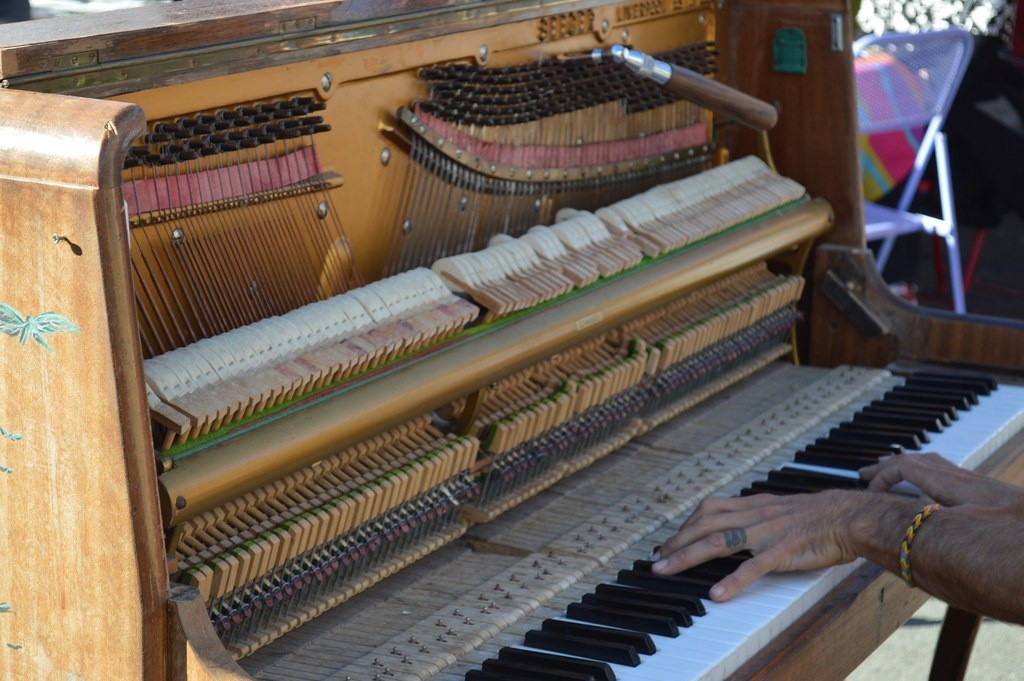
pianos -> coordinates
[1,1,1024,679]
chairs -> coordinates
[854,25,976,312]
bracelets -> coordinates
[899,506,938,600]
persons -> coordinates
[650,452,1024,626]
